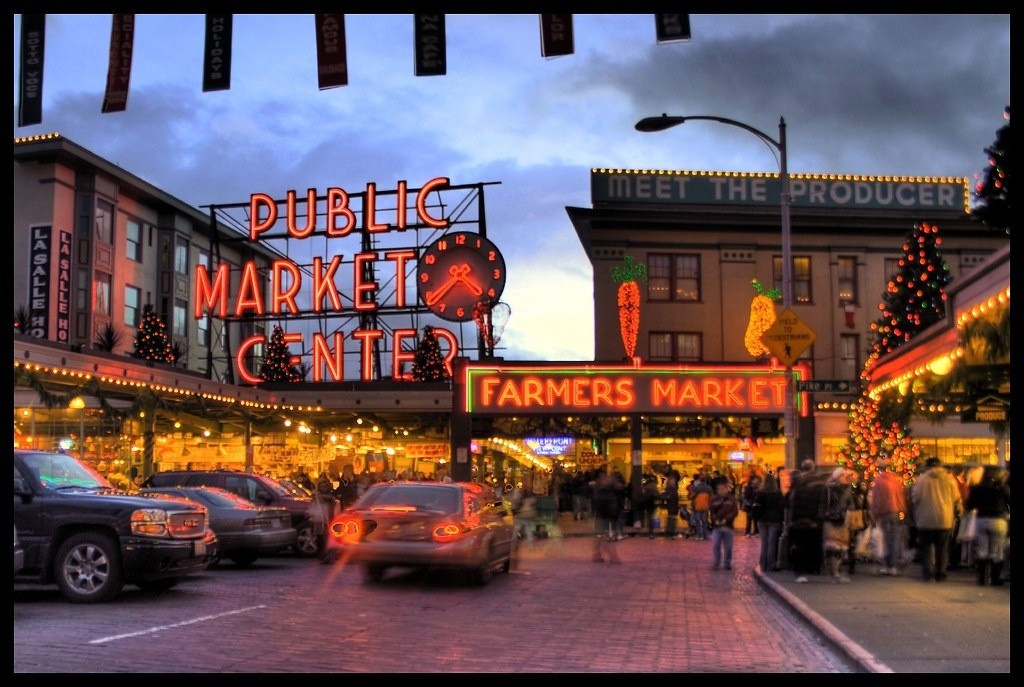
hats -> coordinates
[832,467,846,480]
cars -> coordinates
[138,486,298,570]
[329,483,514,589]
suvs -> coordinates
[15,450,217,603]
[140,470,343,556]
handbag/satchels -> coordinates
[956,509,977,542]
[855,524,886,560]
[846,508,864,530]
[823,523,849,552]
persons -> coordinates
[550,462,862,583]
[910,458,1011,586]
[872,459,904,575]
[300,465,448,535]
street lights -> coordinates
[634,112,793,470]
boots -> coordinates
[990,559,1004,586]
[976,557,989,586]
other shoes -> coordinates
[794,574,808,583]
[722,562,732,571]
[710,565,719,571]
[935,573,947,582]
[878,566,903,577]
[923,574,932,581]
[833,572,840,578]
